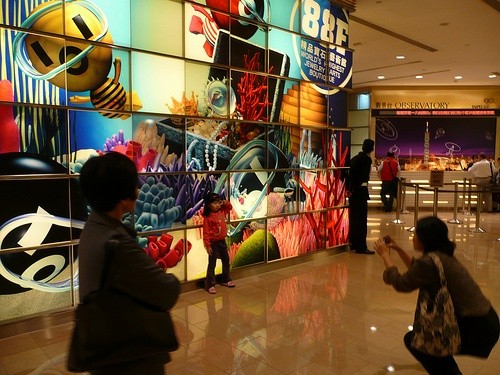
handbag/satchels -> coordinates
[67,234,180,372]
[412,251,461,355]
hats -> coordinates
[363,139,374,150]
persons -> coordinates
[378,151,400,213]
[373,216,500,375]
[203,192,236,294]
[346,139,375,254]
[77,150,179,375]
[468,153,500,212]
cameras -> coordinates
[383,235,390,245]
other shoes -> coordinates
[209,287,216,293]
[356,249,375,254]
[222,281,235,286]
[350,245,356,250]
